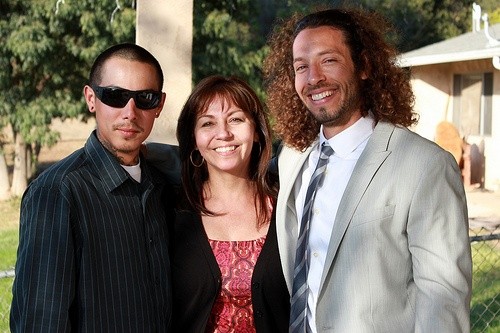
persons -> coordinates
[266,7,472,333]
[177,75,292,333]
[10,44,184,333]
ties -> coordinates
[289,140,334,333]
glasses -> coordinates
[92,84,162,109]
